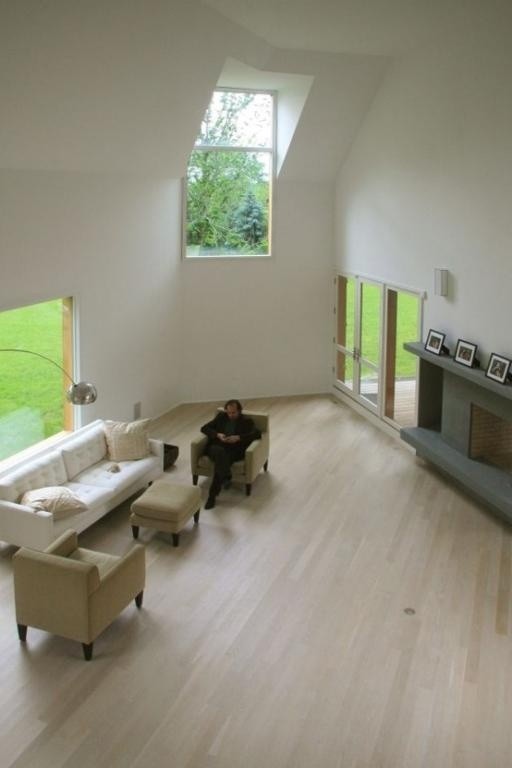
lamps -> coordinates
[434,266,449,298]
[1,348,102,406]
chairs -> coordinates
[10,528,148,662]
[189,403,271,496]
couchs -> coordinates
[0,418,178,552]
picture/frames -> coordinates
[451,337,478,368]
[424,328,446,356]
[484,352,511,384]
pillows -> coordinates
[102,416,154,464]
[19,487,90,519]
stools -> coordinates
[130,479,202,546]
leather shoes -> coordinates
[205,496,215,509]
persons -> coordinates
[199,399,261,510]
